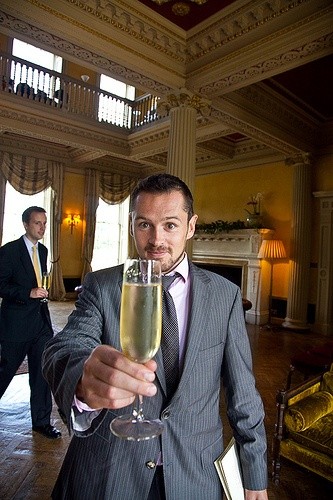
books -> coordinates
[214,436,244,500]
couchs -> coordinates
[270,364,333,486]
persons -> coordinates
[0,206,62,439]
[40,174,269,500]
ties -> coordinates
[32,246,42,288]
[156,274,179,398]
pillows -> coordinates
[284,391,333,432]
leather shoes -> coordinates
[32,424,61,439]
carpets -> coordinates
[14,299,76,374]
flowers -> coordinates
[244,192,264,215]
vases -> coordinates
[244,215,263,227]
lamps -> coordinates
[81,75,90,82]
[133,110,141,126]
[257,240,286,330]
[65,212,80,234]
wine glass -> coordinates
[109,258,164,442]
[40,271,51,302]
